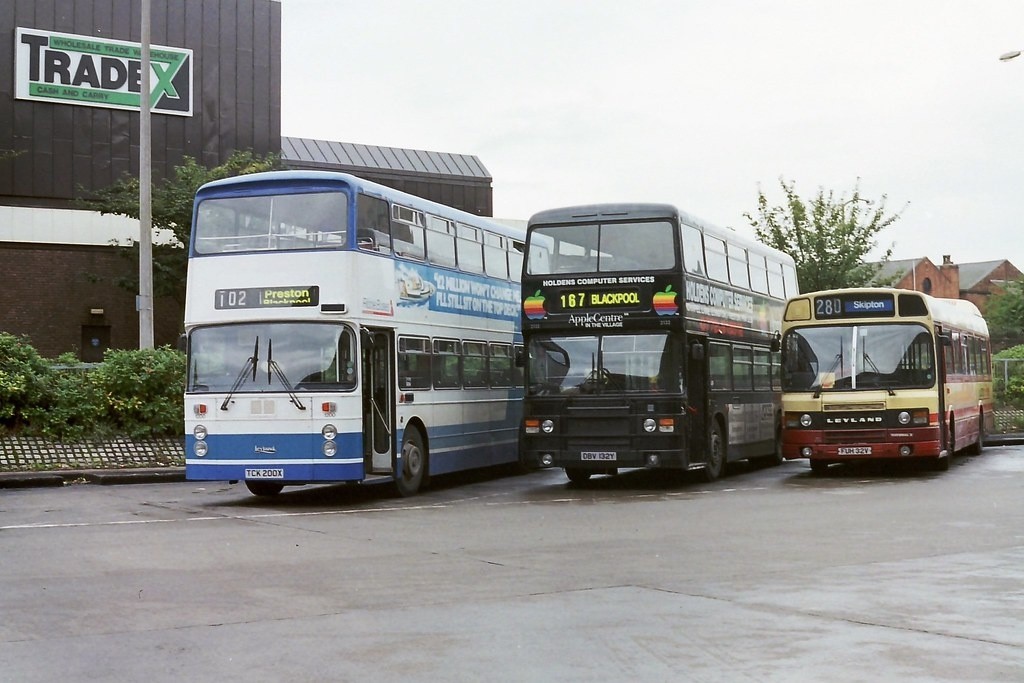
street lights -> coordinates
[840,197,876,239]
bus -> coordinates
[770,286,995,472]
[183,170,551,498]
[517,201,802,487]
